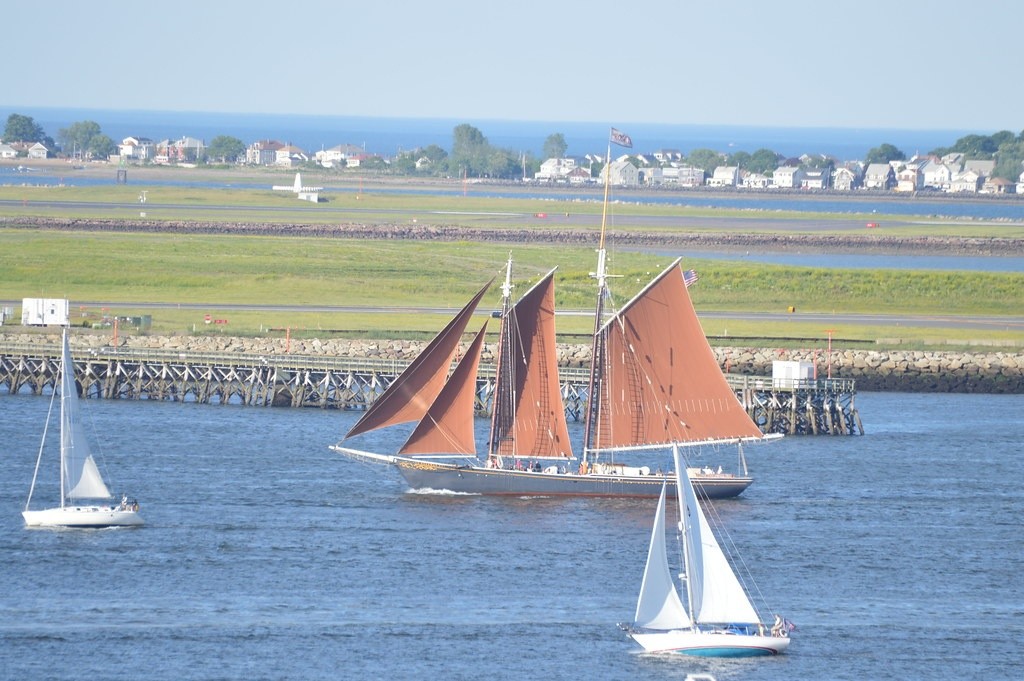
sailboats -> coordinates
[327,250,787,495]
[632,440,795,658]
[22,329,145,526]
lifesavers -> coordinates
[779,629,788,638]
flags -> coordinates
[609,127,632,148]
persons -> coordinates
[534,460,542,472]
[770,613,783,637]
